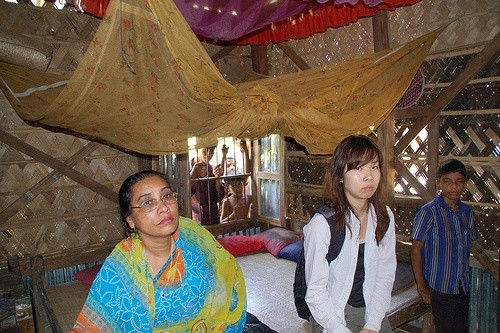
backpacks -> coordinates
[293,202,347,322]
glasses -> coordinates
[131,192,178,213]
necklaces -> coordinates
[146,236,174,298]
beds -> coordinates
[29,219,435,333]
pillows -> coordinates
[391,259,416,294]
[70,264,101,285]
[216,228,304,264]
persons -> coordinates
[293,135,397,333]
[71,170,247,333]
[189,135,252,225]
[411,159,500,333]
